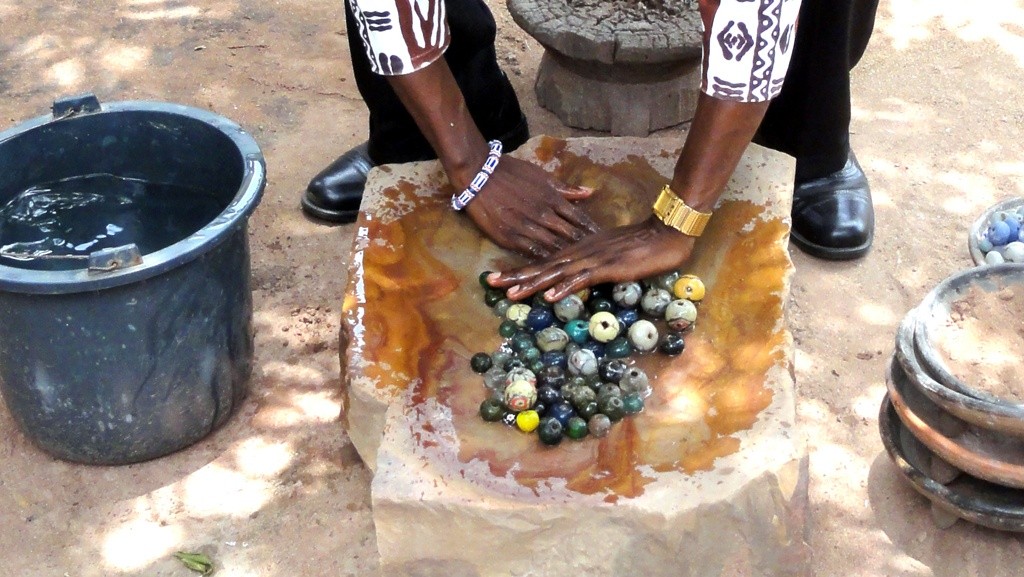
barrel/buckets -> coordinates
[0,93,265,468]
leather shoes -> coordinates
[790,148,874,258]
[301,140,374,223]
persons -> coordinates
[300,0,882,303]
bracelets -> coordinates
[450,139,504,211]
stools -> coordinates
[507,0,706,136]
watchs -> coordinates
[652,183,712,237]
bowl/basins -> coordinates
[876,195,1023,536]
